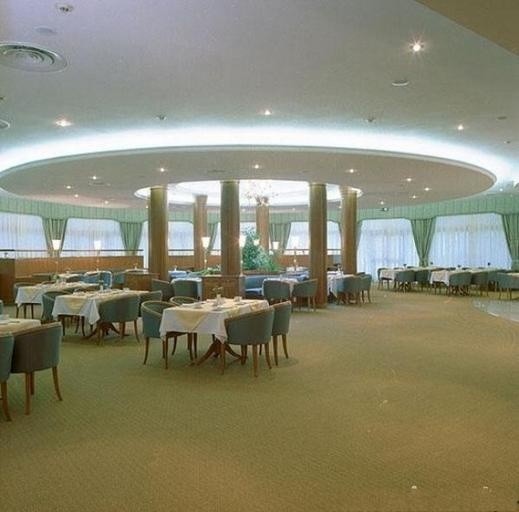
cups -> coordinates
[0,313,9,324]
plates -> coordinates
[182,292,248,311]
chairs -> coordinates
[169,295,200,357]
[222,306,272,377]
[11,263,318,326]
[261,300,292,366]
[326,263,519,307]
[1,332,17,421]
[140,300,173,366]
[11,322,64,416]
[82,294,139,346]
